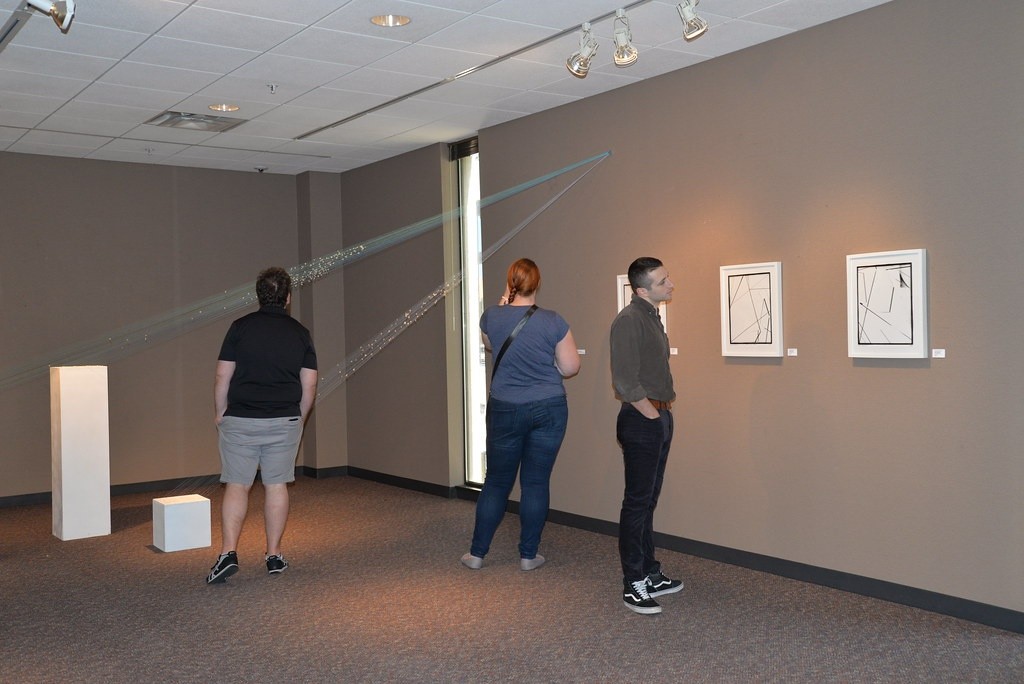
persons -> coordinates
[461,258,580,571]
[206,267,317,584]
[610,257,683,614]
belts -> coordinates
[649,400,672,410]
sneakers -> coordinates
[206,550,239,584]
[622,577,662,614]
[645,570,684,598]
[265,552,291,573]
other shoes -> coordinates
[521,554,545,570]
[461,552,483,569]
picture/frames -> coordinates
[616,274,667,333]
[720,262,783,358]
[846,248,928,358]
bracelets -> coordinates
[501,296,506,300]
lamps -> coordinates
[26,0,76,30]
[566,21,597,78]
[612,8,637,67]
[676,0,708,40]
